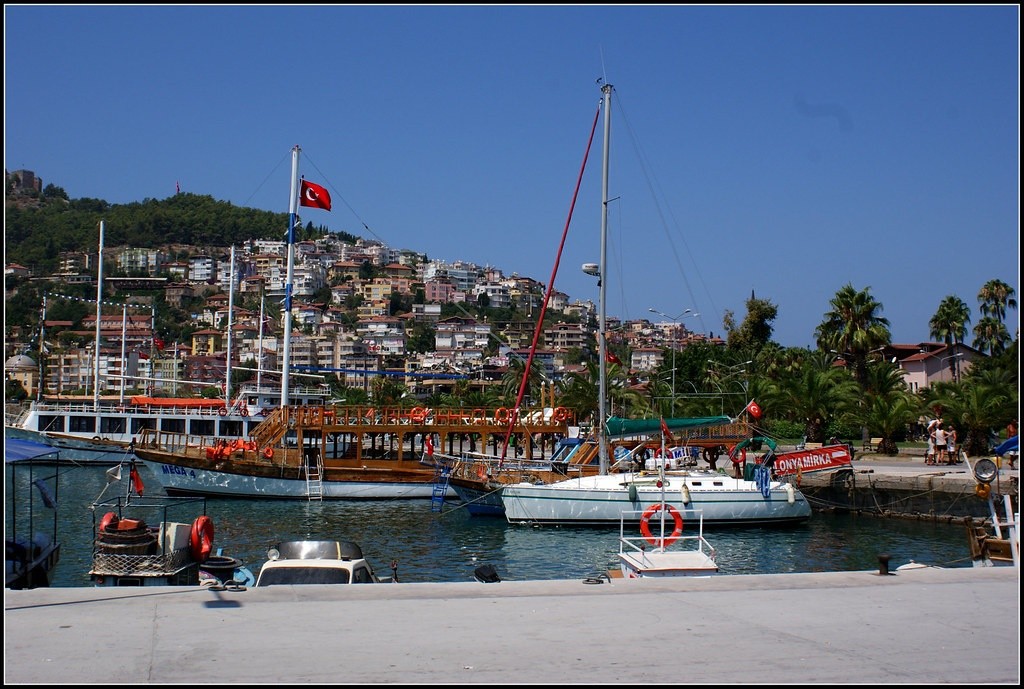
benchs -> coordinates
[924,444,962,463]
[804,442,823,450]
[862,437,884,452]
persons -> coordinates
[1007,418,1019,469]
[213,436,239,449]
[150,437,157,447]
[924,409,957,465]
[130,436,136,450]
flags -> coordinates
[131,470,144,493]
[139,349,151,360]
[605,349,623,367]
[661,419,676,440]
[747,401,762,418]
[298,177,331,210]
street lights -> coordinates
[920,350,964,382]
[649,308,701,418]
[708,360,753,392]
[657,369,677,383]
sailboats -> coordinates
[482,80,814,527]
[10,139,461,499]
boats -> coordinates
[254,539,398,587]
[433,413,751,516]
[608,408,722,579]
[87,437,257,587]
[6,439,63,591]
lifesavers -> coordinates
[102,437,110,442]
[93,436,102,446]
[729,445,746,463]
[702,448,720,463]
[190,516,214,565]
[633,449,650,466]
[410,406,426,423]
[640,504,681,547]
[495,407,509,423]
[218,407,228,417]
[264,447,274,457]
[655,448,674,466]
[261,408,269,417]
[555,406,568,421]
[240,408,248,417]
[472,409,486,423]
